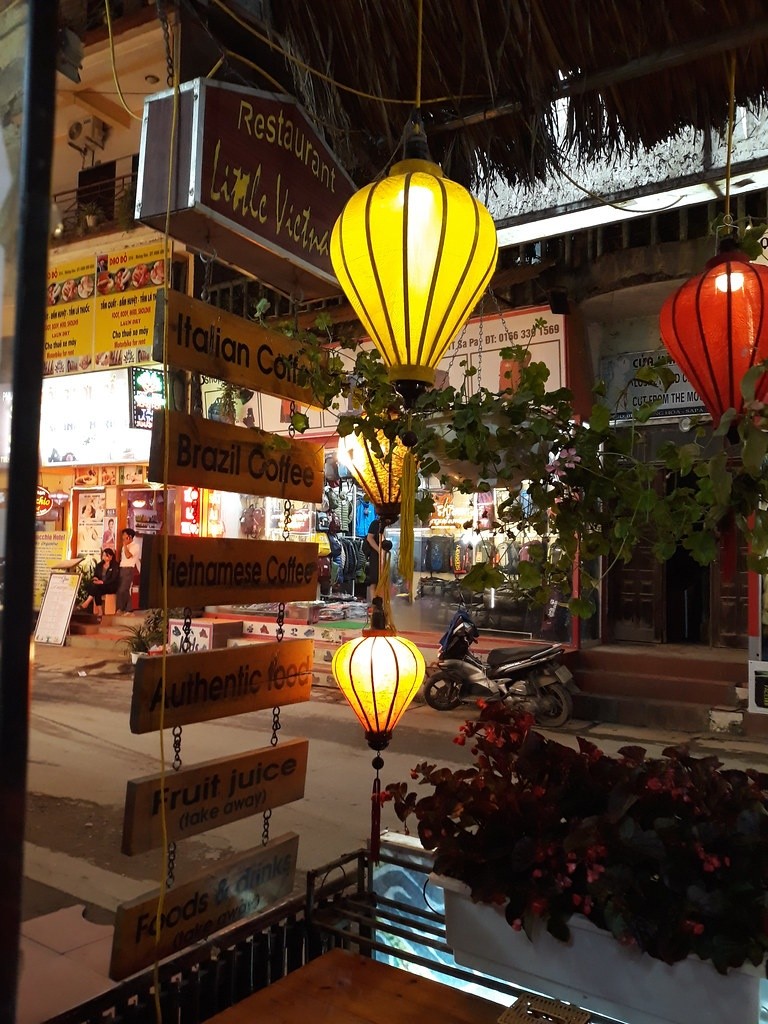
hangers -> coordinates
[336,536,367,542]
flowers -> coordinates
[388,701,767,974]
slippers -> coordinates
[96,613,103,624]
[76,605,88,611]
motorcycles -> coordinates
[422,592,580,727]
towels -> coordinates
[438,608,479,651]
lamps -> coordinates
[331,598,428,774]
[336,405,423,523]
[331,3,496,389]
[656,2,768,425]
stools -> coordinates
[93,594,116,615]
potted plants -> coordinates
[74,200,112,235]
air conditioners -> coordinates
[68,116,108,148]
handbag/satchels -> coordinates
[447,579,541,637]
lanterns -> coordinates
[330,158,499,605]
[331,610,425,866]
[659,249,768,444]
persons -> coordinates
[361,515,399,614]
[74,548,119,622]
[114,528,140,617]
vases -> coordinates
[424,854,763,1024]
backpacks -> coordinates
[327,532,342,557]
[329,557,340,586]
[518,540,541,563]
[311,532,331,556]
[324,451,340,481]
[338,460,351,478]
[316,510,329,531]
[315,494,329,512]
[326,510,340,533]
[325,488,339,509]
[317,557,332,584]
[497,540,518,574]
[239,504,255,534]
[423,536,449,573]
[450,534,472,574]
[208,397,236,425]
[519,490,533,518]
[475,537,495,563]
[546,538,565,567]
[252,507,265,538]
